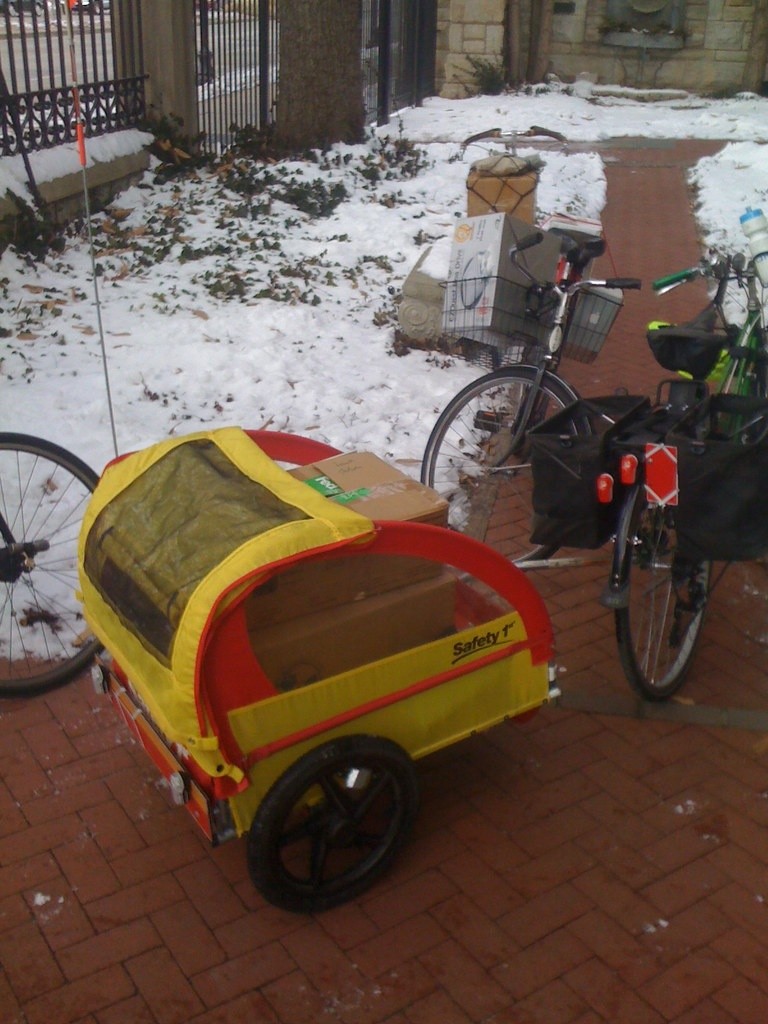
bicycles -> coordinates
[421,227,768,704]
[0,431,110,697]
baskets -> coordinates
[439,276,625,372]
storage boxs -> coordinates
[241,449,449,633]
[544,212,601,289]
[563,285,624,352]
[249,573,461,694]
[442,213,562,349]
[466,166,539,225]
